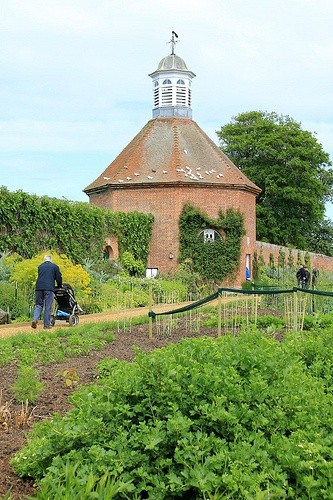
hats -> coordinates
[300,264,304,268]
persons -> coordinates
[296,263,319,289]
[67,289,84,312]
[246,267,251,281]
[31,254,64,329]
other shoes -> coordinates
[44,326,52,329]
[32,320,37,328]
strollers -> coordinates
[50,283,80,326]
